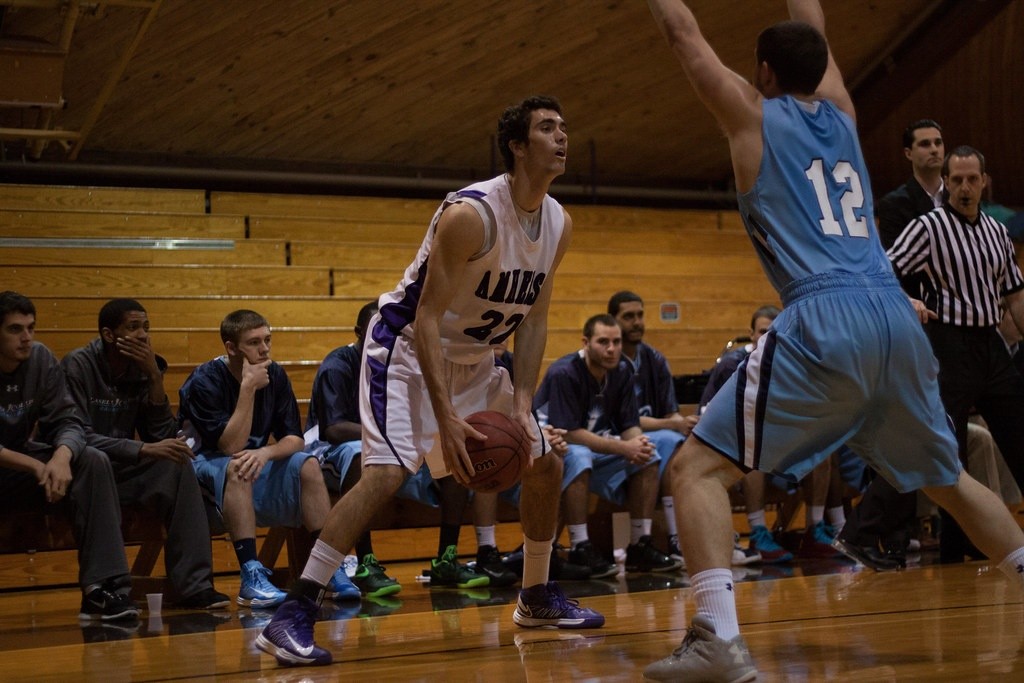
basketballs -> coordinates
[454,410,534,494]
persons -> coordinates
[0,289,139,621]
[61,298,233,611]
[608,290,765,565]
[176,310,369,609]
[644,1,1024,683]
[698,304,880,571]
[304,301,491,598]
[889,124,1024,566]
[479,332,568,587]
[537,313,686,579]
[254,95,610,666]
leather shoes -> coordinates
[837,531,901,570]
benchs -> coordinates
[0,185,784,589]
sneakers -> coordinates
[324,561,361,598]
[77,584,141,620]
[328,590,510,641]
[642,614,758,683]
[514,629,605,667]
[749,531,793,563]
[473,556,517,586]
[354,553,402,596]
[798,522,848,557]
[624,536,682,571]
[235,560,287,608]
[177,586,231,609]
[667,538,686,571]
[731,541,762,565]
[254,595,332,666]
[513,581,604,628]
[421,545,489,589]
[77,619,142,651]
[506,539,620,579]
